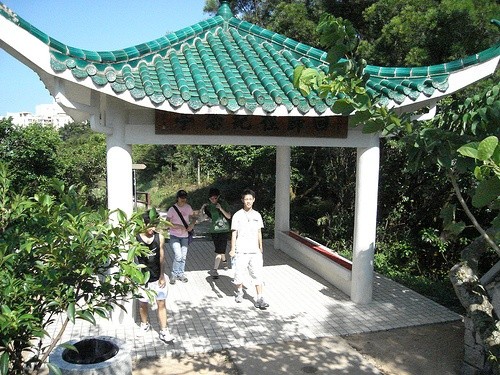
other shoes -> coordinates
[212,269,219,279]
[159,328,175,342]
[223,260,228,269]
[254,298,269,308]
[177,274,188,283]
[136,322,150,337]
[169,275,176,284]
[235,292,243,303]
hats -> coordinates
[178,190,188,199]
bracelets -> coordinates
[191,224,194,228]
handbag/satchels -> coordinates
[187,229,194,244]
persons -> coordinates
[229,189,269,308]
[131,210,175,343]
[164,190,196,284]
[199,188,232,277]
